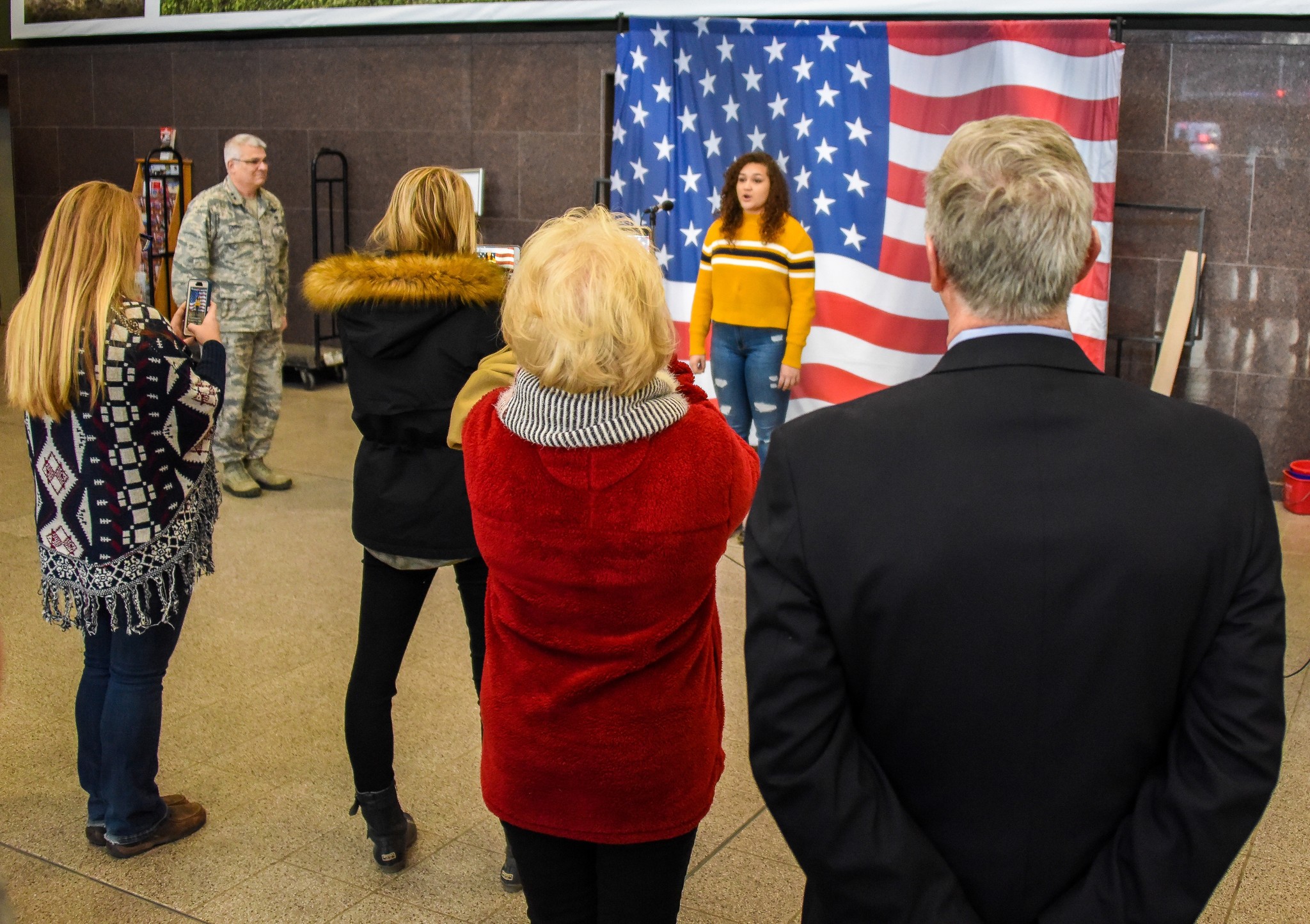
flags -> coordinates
[612,18,1125,452]
[189,287,207,312]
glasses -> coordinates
[233,158,268,165]
[139,232,154,251]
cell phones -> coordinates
[183,278,214,336]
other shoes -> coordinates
[729,522,745,545]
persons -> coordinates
[195,295,201,318]
[6,180,226,858]
[302,166,527,893]
[691,152,814,543]
[171,133,293,497]
[741,119,1286,924]
[460,204,758,924]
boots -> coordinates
[501,842,524,894]
[349,782,417,874]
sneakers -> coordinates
[245,458,292,490]
[222,460,262,498]
[107,802,207,859]
[86,794,188,847]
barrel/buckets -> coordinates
[1283,461,1310,515]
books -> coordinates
[135,163,182,303]
[160,126,176,161]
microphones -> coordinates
[643,201,673,214]
